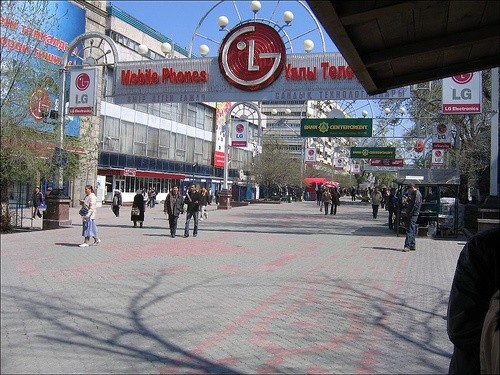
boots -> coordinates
[139,222,143,228]
[132,222,137,228]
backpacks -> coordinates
[479,288,500,375]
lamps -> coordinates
[362,110,368,117]
[161,41,171,59]
[138,44,148,61]
[251,0,261,17]
[285,109,291,118]
[400,108,405,116]
[283,10,293,27]
[218,15,229,33]
[272,108,277,117]
[384,107,391,117]
[198,45,208,57]
[303,39,315,53]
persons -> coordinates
[131,186,158,228]
[371,187,383,218]
[111,188,123,217]
[184,184,201,237]
[281,184,304,202]
[402,182,422,252]
[164,184,184,238]
[388,188,396,229]
[447,227,500,375]
[79,184,98,246]
[316,182,358,215]
[370,185,398,210]
[199,186,220,219]
[29,185,53,219]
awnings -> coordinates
[308,0,500,95]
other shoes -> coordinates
[200,218,203,221]
[92,240,101,245]
[79,243,89,247]
[184,233,189,237]
[172,232,175,238]
[37,217,42,219]
[193,234,196,237]
[204,212,208,219]
[403,248,409,252]
[32,217,34,220]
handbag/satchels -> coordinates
[132,208,140,215]
[79,208,89,216]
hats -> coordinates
[35,186,40,189]
[48,187,52,191]
